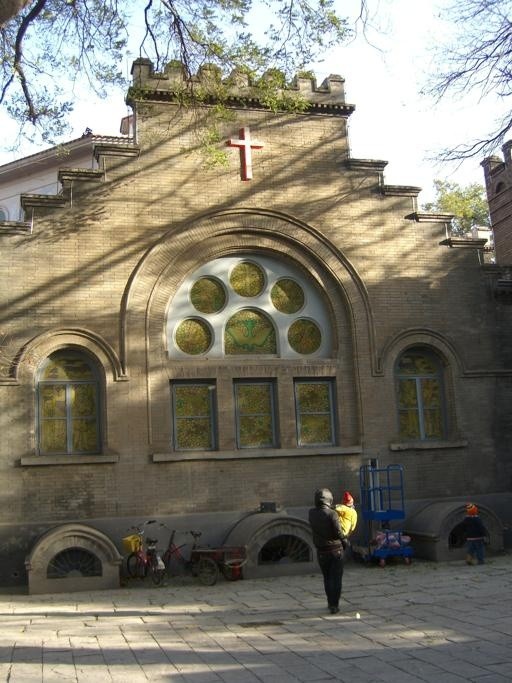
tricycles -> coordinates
[157,522,247,587]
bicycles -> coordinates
[123,518,167,584]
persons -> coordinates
[332,488,357,535]
[460,501,487,566]
[309,486,350,614]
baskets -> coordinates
[122,535,143,552]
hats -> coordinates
[343,492,354,507]
[466,503,478,515]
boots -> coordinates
[466,554,474,565]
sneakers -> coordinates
[331,607,339,612]
